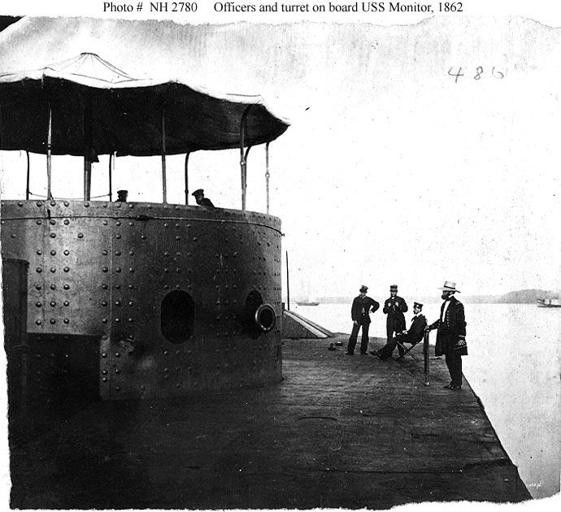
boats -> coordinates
[294,299,320,307]
[535,297,560,309]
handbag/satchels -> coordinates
[452,344,468,356]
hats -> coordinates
[359,285,368,293]
[413,302,422,309]
[117,190,127,194]
[191,189,204,196]
[437,280,461,294]
[389,285,398,292]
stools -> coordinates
[394,338,420,362]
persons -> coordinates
[371,302,427,361]
[425,282,467,390]
[384,286,408,359]
[115,190,128,202]
[345,286,380,356]
[193,189,216,208]
